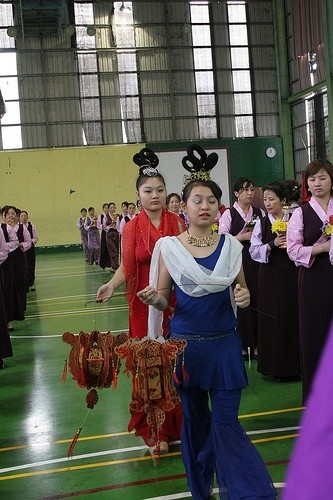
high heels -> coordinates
[241,347,249,361]
[252,347,259,360]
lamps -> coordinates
[119,2,132,14]
[183,24,191,34]
[64,26,74,35]
[7,27,17,37]
[86,26,96,36]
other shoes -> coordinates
[148,442,169,455]
[0,358,4,369]
[7,323,14,332]
[31,285,35,291]
[110,267,115,274]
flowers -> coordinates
[323,215,333,237]
[271,219,287,248]
[212,224,217,232]
[244,214,259,232]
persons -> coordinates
[135,144,278,500]
[0,205,39,369]
[77,159,333,405]
[96,147,187,456]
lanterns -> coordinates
[61,331,130,458]
[114,335,188,465]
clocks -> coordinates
[266,147,276,157]
